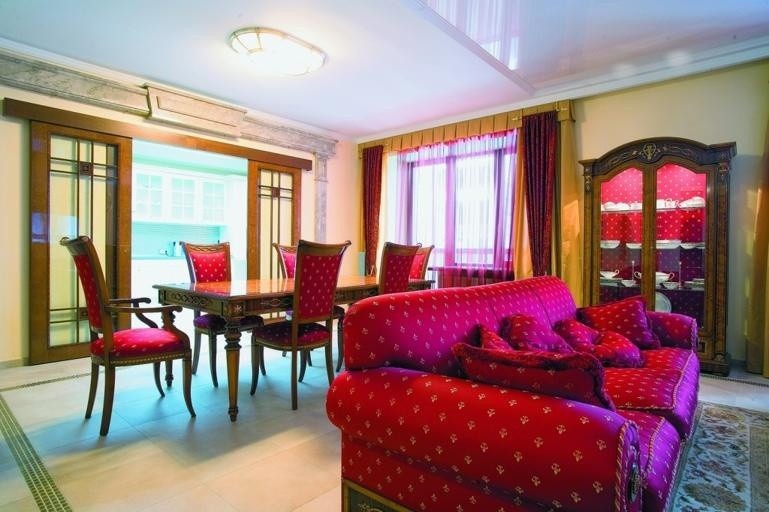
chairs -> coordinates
[272,239,351,373]
[57,234,200,436]
[244,239,337,411]
[177,237,268,390]
[410,244,438,281]
[377,240,423,295]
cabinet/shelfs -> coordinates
[577,136,738,376]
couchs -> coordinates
[323,274,702,512]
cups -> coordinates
[679,196,706,207]
[601,198,680,211]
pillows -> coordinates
[453,292,665,413]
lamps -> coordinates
[227,25,326,76]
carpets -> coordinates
[659,400,769,512]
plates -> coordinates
[655,292,672,314]
[599,278,623,282]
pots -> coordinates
[634,270,674,286]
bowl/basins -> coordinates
[621,279,636,287]
[627,243,642,248]
[600,241,621,249]
[684,278,704,290]
[600,270,618,279]
[656,239,680,249]
[662,281,679,289]
[682,244,695,250]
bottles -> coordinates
[358,251,365,277]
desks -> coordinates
[149,272,439,424]
